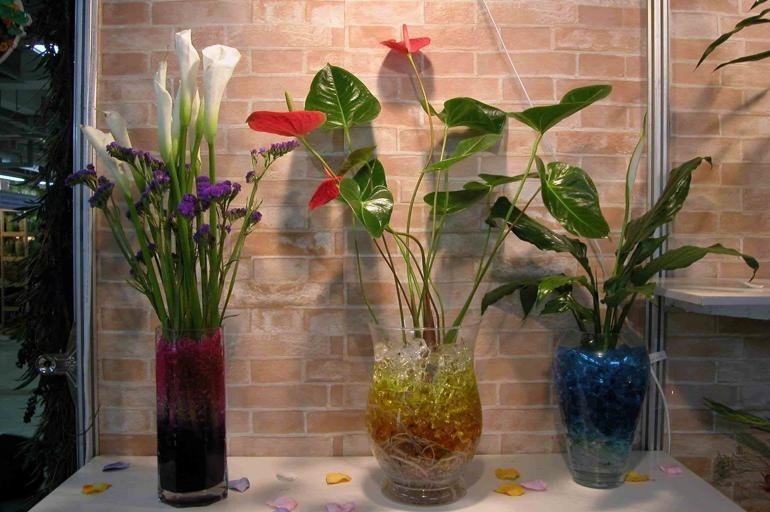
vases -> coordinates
[369,318,478,506]
[151,325,229,504]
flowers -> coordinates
[64,29,310,324]
[245,22,610,343]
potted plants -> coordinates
[463,92,757,494]
